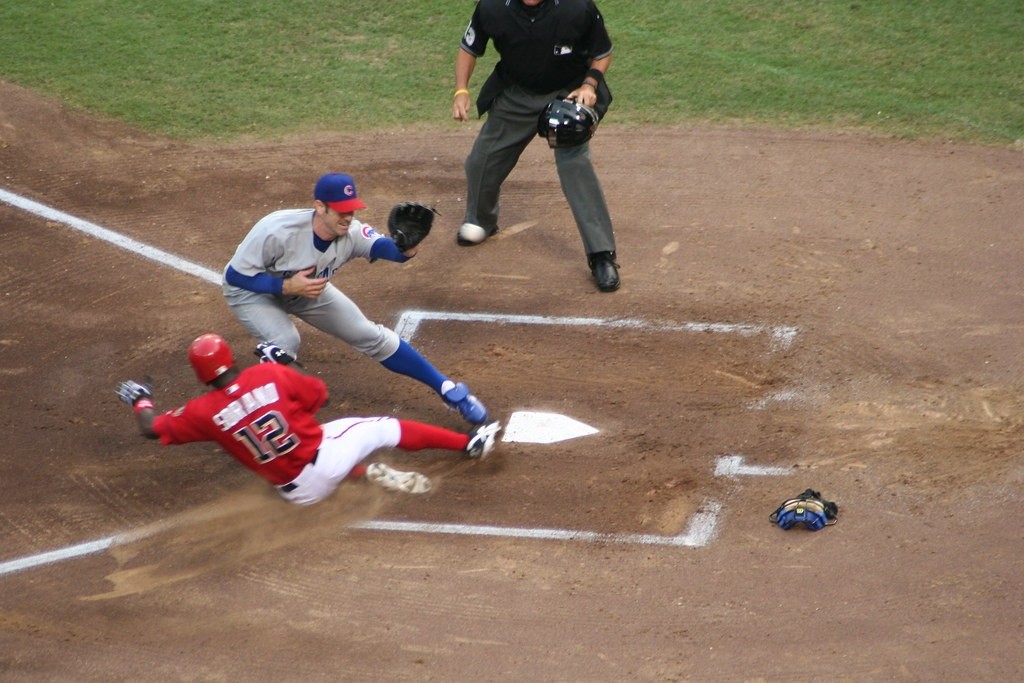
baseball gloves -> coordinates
[769,488,838,532]
[387,202,433,250]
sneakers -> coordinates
[442,382,486,424]
[464,420,501,460]
[366,463,432,494]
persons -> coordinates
[113,333,504,507]
[222,172,487,426]
[452,0,620,291]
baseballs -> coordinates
[459,223,486,244]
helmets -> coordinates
[537,96,599,149]
[189,334,236,386]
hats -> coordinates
[314,171,367,213]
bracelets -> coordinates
[134,399,154,413]
[585,68,603,83]
[582,81,597,89]
[454,89,470,98]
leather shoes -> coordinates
[457,223,498,246]
[591,255,620,291]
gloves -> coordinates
[116,380,152,406]
[253,341,294,365]
